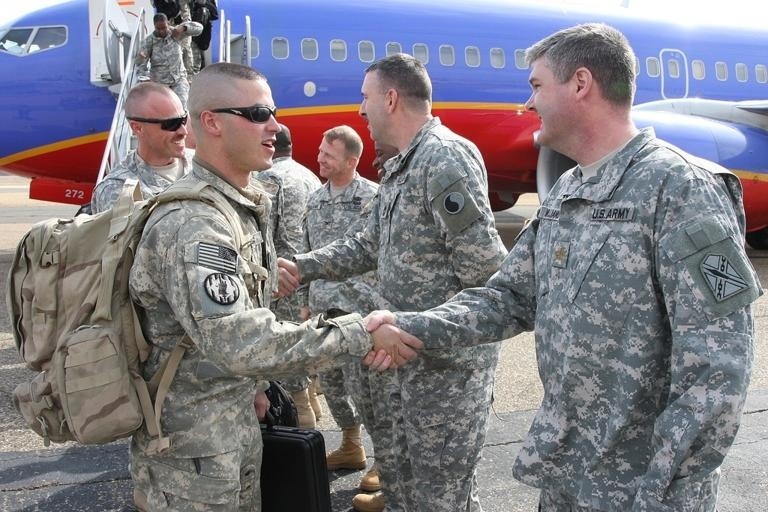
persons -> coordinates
[126,63,422,512]
[352,138,400,512]
[276,52,509,512]
[256,124,323,431]
[134,0,218,147]
[293,125,381,492]
[90,82,300,301]
[362,22,765,512]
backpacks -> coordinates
[5,181,243,446]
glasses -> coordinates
[126,111,188,131]
[211,106,276,124]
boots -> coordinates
[308,377,323,417]
[292,389,317,430]
[327,426,366,471]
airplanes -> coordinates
[0,0,768,251]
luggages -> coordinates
[261,381,331,512]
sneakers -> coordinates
[352,492,385,512]
[361,464,381,491]
